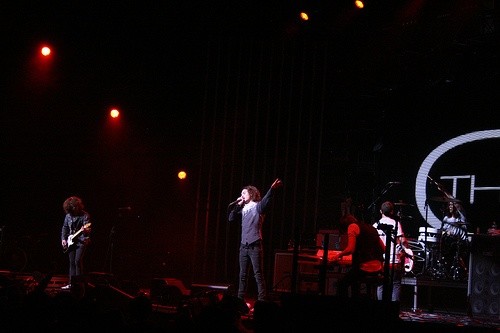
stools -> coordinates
[364,276,384,303]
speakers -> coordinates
[465,235,500,318]
[273,253,374,299]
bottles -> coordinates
[492,221,496,233]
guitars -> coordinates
[63,223,91,255]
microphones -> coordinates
[230,199,242,205]
[389,182,399,184]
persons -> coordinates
[372,202,405,301]
[441,201,467,236]
[333,214,382,296]
[228,179,282,302]
[61,196,92,289]
[0,291,244,333]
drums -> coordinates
[417,227,442,243]
[403,241,426,277]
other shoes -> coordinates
[61,284,72,289]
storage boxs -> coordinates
[317,234,338,247]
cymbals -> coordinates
[427,197,463,202]
[394,203,415,206]
[450,222,470,225]
